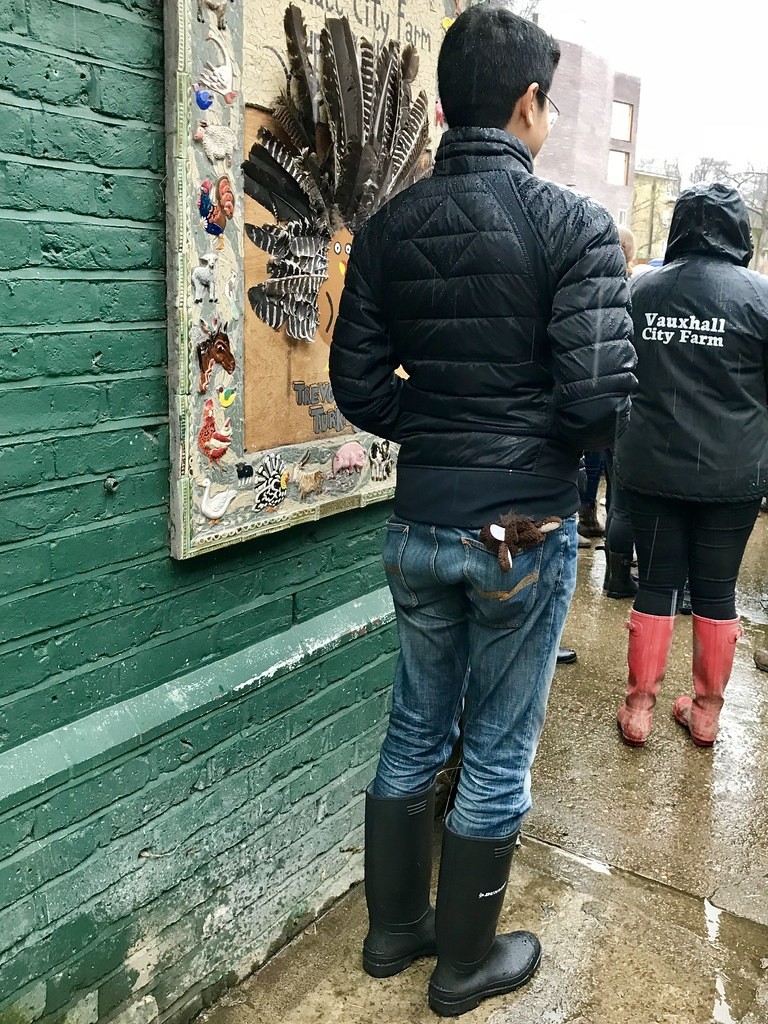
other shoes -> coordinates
[754,649,768,674]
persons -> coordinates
[328,2,639,1017]
[610,182,768,746]
[553,224,768,672]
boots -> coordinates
[364,776,434,977]
[576,467,605,539]
[423,808,540,1018]
[615,606,675,746]
[602,546,639,600]
[672,611,745,749]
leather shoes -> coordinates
[555,649,582,666]
[578,532,591,550]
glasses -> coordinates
[535,84,565,135]
